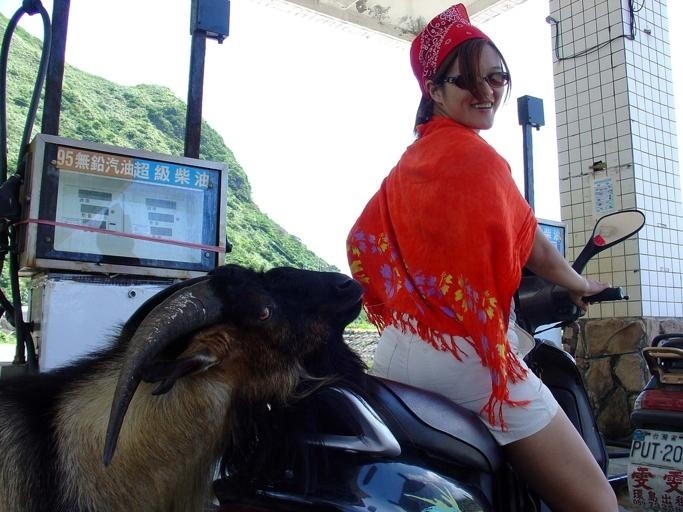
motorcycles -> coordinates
[627,332,683,512]
[208,209,647,512]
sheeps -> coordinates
[0,263,363,512]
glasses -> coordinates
[442,71,510,88]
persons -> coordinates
[346,1,620,512]
